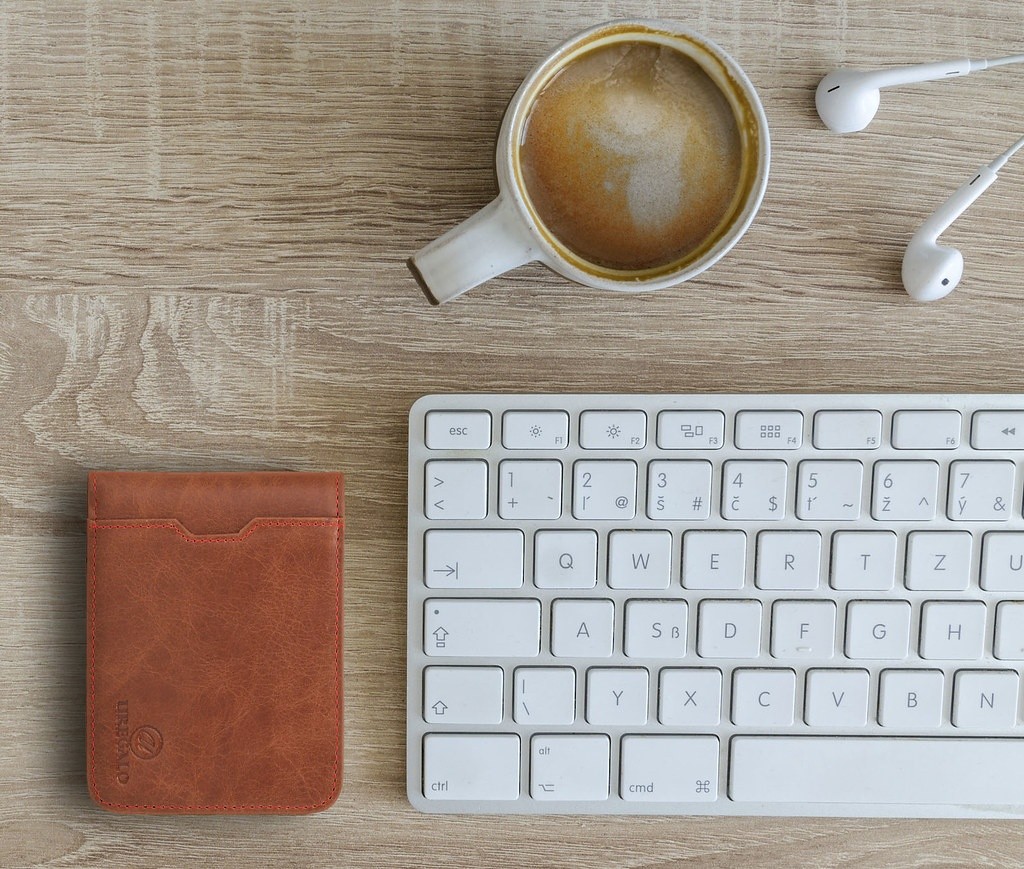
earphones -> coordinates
[901,153,1007,301]
[815,57,988,132]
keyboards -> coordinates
[405,395,1024,823]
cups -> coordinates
[399,13,774,308]
